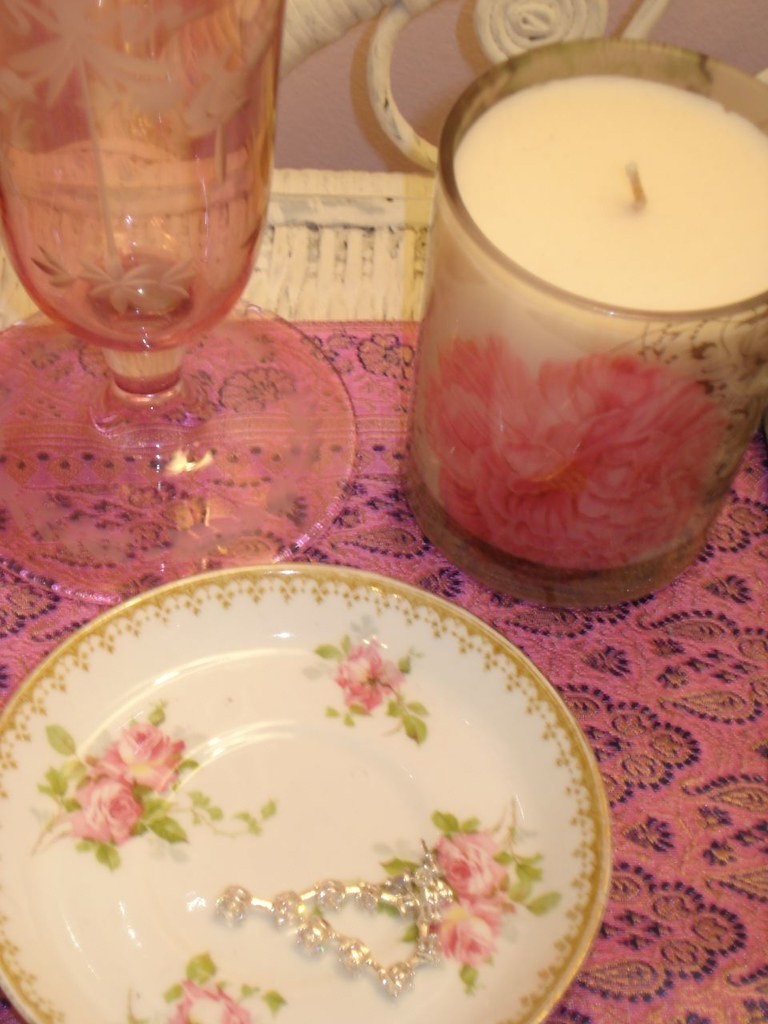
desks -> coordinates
[0,169,768,1022]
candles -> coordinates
[415,74,767,577]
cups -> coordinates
[405,37,767,607]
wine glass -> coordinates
[0,0,362,605]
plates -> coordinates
[0,564,616,1024]
